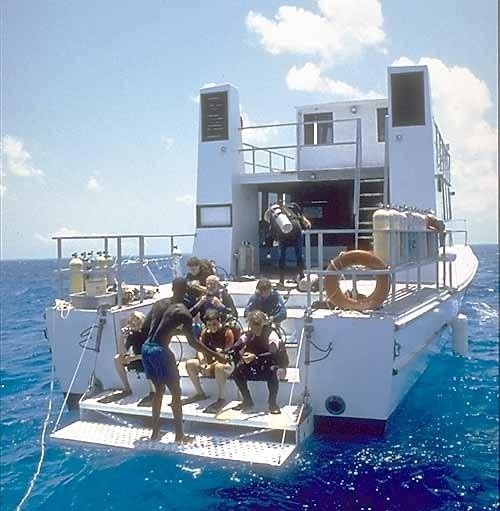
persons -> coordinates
[231,309,287,416]
[185,309,236,415]
[189,275,239,339]
[97,311,158,409]
[139,277,231,448]
[269,200,312,289]
[242,279,288,331]
[184,255,216,307]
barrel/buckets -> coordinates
[84,279,107,296]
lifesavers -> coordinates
[323,249,391,310]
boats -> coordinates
[42,62,481,471]
